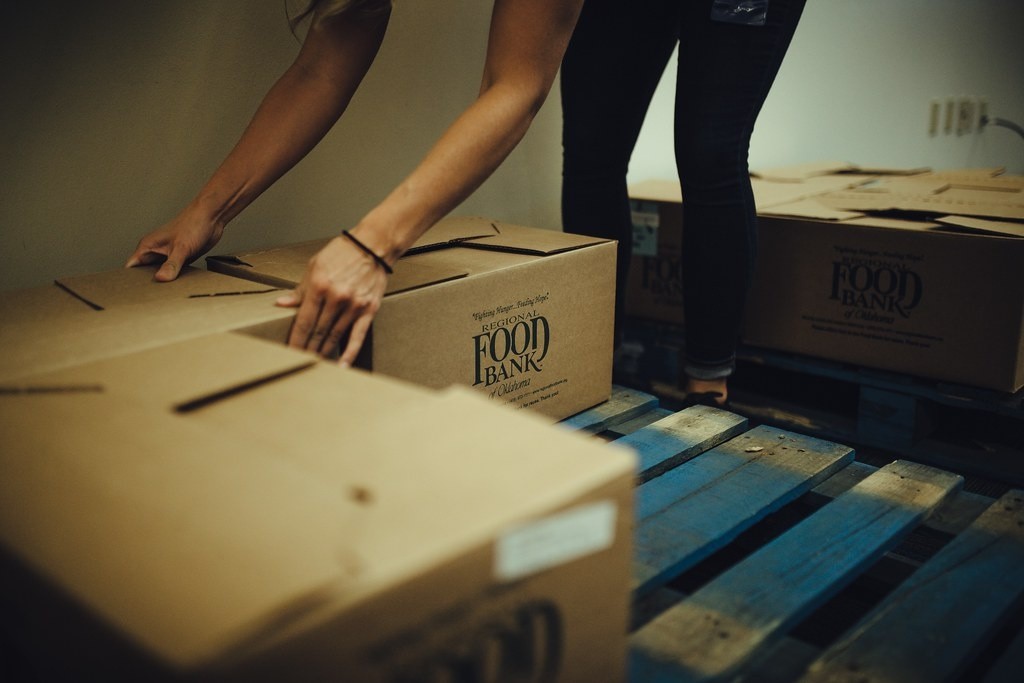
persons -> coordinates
[127,0,807,408]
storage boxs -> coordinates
[0,330,639,683]
[623,163,1024,394]
[0,262,340,384]
[204,212,619,420]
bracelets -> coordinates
[343,230,394,274]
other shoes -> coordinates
[681,391,729,410]
